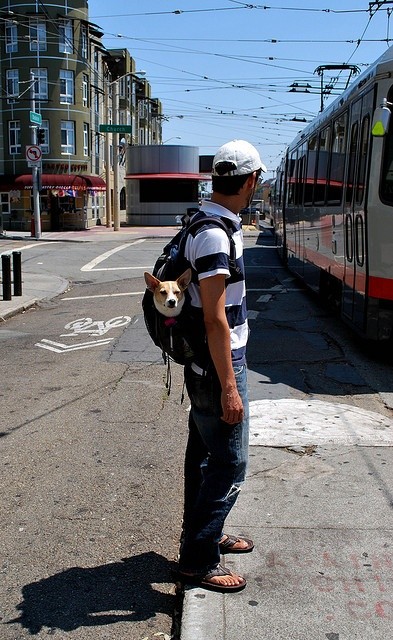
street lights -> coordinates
[162,136,180,147]
[155,115,184,144]
[105,70,146,231]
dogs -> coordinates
[141,262,193,320]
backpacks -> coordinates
[141,210,237,405]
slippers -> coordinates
[179,564,247,592]
[219,534,253,553]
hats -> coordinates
[212,140,267,176]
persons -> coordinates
[175,138,266,593]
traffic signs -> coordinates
[26,146,41,163]
[30,111,43,126]
[99,124,131,135]
